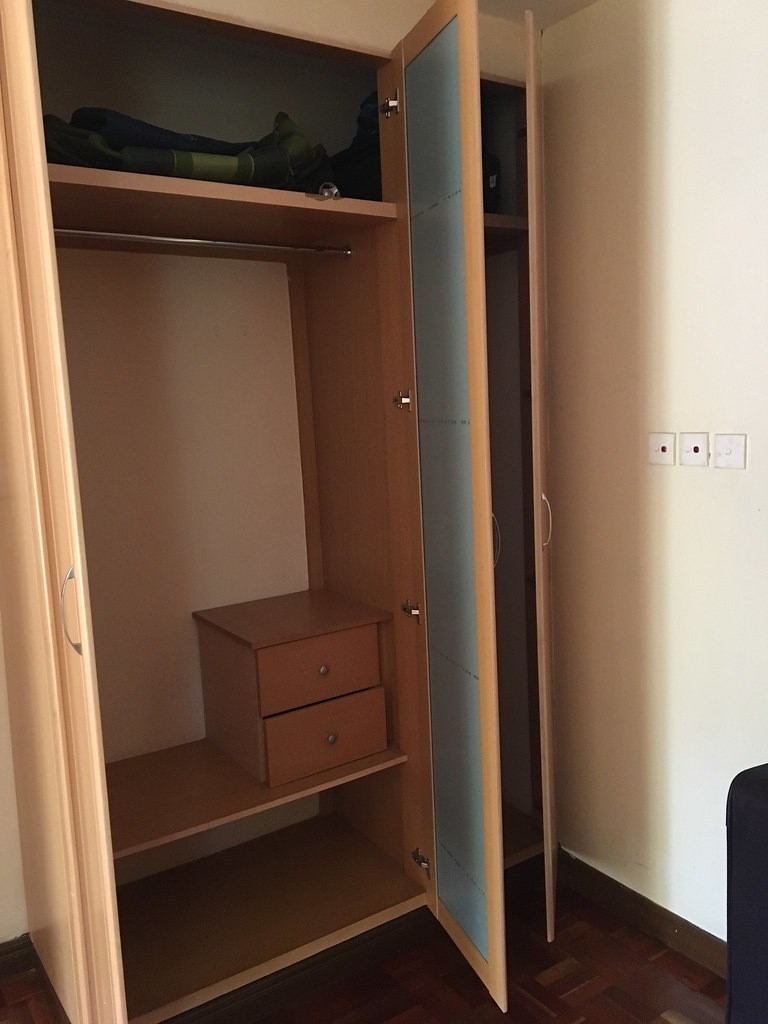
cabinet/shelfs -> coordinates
[0,0,513,1024]
[185,583,387,785]
